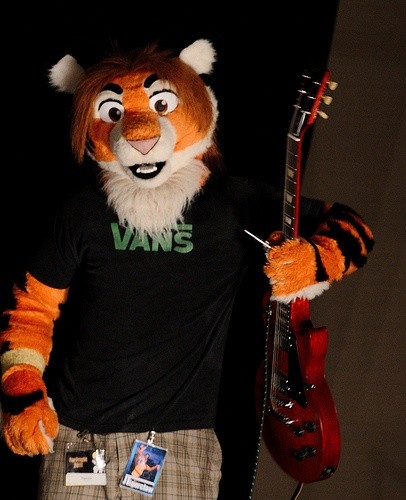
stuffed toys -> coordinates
[0,39,375,500]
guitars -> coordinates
[261,67,339,485]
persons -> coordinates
[131,445,160,477]
